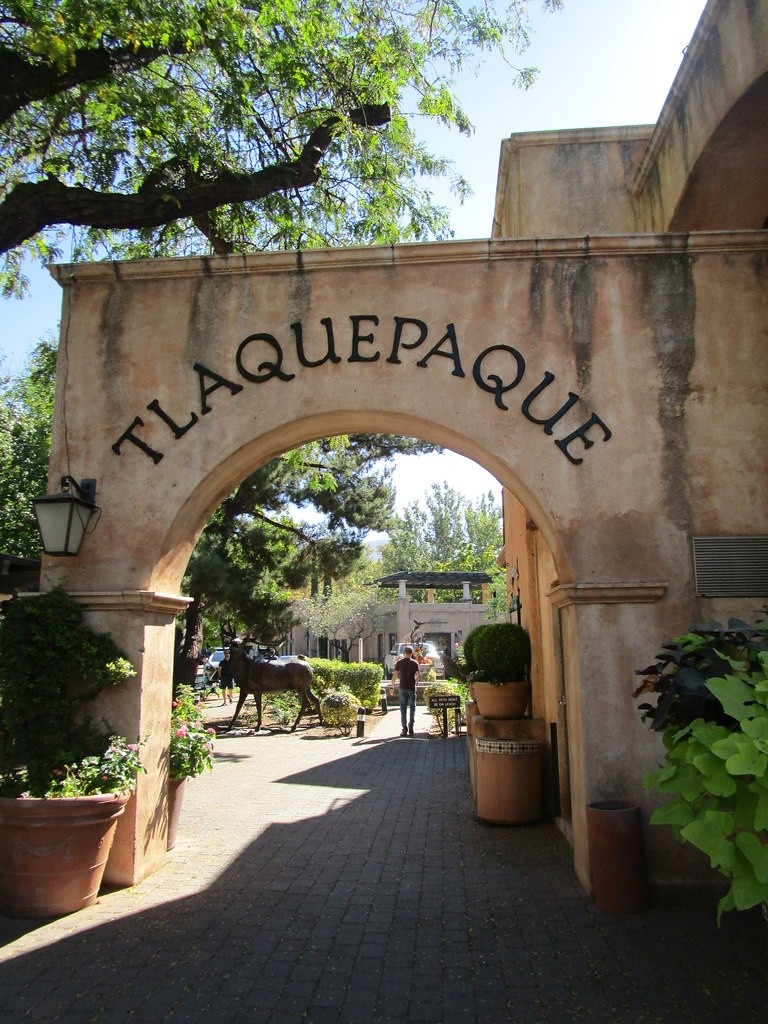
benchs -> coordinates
[195,676,219,701]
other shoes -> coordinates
[409,723,414,736]
[221,702,227,706]
[400,725,408,737]
[229,698,232,704]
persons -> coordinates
[389,646,421,737]
[264,647,278,660]
[297,653,306,660]
[218,650,232,706]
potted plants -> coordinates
[465,624,487,703]
[472,623,531,719]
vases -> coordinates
[166,772,188,851]
[420,664,432,674]
[0,772,131,917]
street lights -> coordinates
[305,630,310,650]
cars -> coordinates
[195,668,212,690]
[384,643,445,681]
[203,651,225,668]
[202,652,210,661]
[280,655,298,661]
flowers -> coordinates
[0,719,158,801]
[170,683,216,779]
[413,648,432,664]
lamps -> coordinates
[30,472,96,555]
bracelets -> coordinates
[390,688,394,689]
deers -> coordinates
[213,615,324,733]
